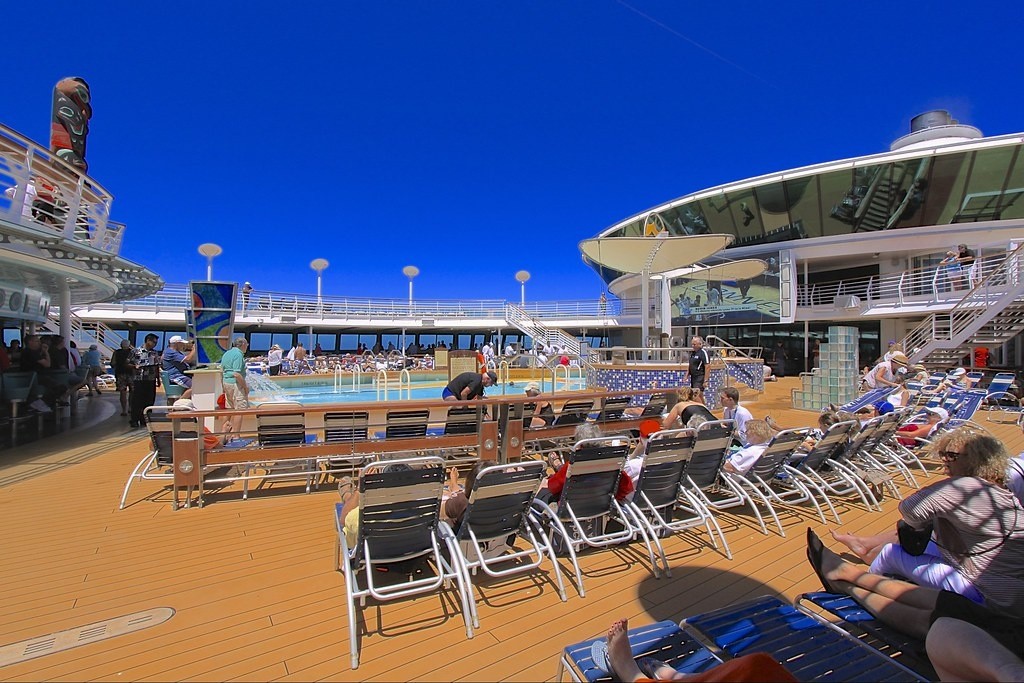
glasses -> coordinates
[151,340,158,344]
[939,450,968,462]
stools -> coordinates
[0,364,103,442]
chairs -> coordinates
[316,350,1024,682]
[119,405,254,510]
[241,400,317,500]
[244,354,315,377]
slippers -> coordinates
[766,415,776,426]
[806,527,835,593]
[590,639,672,683]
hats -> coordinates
[874,400,894,415]
[953,367,966,376]
[523,382,540,391]
[169,335,189,344]
[891,355,908,368]
[486,371,498,386]
[640,420,661,438]
[90,345,97,349]
[171,399,197,410]
[914,365,924,370]
[924,406,948,418]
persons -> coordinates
[242,282,255,310]
[442,371,498,421]
[894,178,930,231]
[804,525,1023,661]
[829,528,899,566]
[219,337,250,439]
[837,166,878,215]
[149,398,234,452]
[606,618,1024,683]
[866,426,1024,616]
[599,341,607,364]
[621,338,1024,491]
[954,243,979,288]
[549,418,662,503]
[4,172,110,240]
[509,381,515,387]
[523,382,556,427]
[0,332,197,428]
[439,460,505,536]
[506,422,634,513]
[249,339,570,375]
[670,256,780,324]
[940,250,963,291]
[599,292,607,316]
[337,463,416,551]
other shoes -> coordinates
[85,393,94,397]
[96,390,101,394]
[131,420,146,427]
[121,413,127,416]
[30,399,49,412]
[127,411,132,413]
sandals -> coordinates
[337,475,355,503]
[548,452,564,472]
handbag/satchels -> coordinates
[897,519,933,557]
